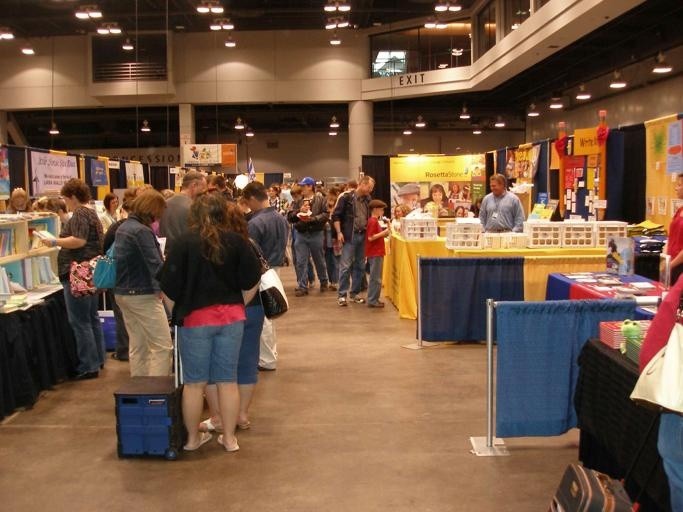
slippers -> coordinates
[184,418,250,452]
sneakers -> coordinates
[296,285,387,307]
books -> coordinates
[0,210,63,314]
[559,270,670,369]
[625,220,668,257]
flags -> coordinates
[247,160,256,182]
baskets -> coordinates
[400,216,629,247]
[601,319,649,355]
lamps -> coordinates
[402,0,681,136]
[71,0,354,50]
[21,37,34,56]
[0,25,15,40]
[138,113,341,137]
[48,121,59,135]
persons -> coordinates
[399,182,470,217]
[665,173,683,289]
[99,170,290,452]
[289,174,411,308]
[629,271,683,511]
[478,172,524,235]
[469,198,484,219]
[5,179,130,381]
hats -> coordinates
[368,199,388,208]
[297,176,316,186]
[398,183,420,196]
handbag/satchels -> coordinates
[93,241,118,289]
[629,321,683,412]
[248,236,289,320]
[69,255,103,299]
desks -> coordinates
[545,272,683,326]
[0,286,81,421]
[387,217,663,344]
[570,337,671,512]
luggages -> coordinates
[545,405,661,511]
[113,323,184,461]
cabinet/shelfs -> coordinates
[0,211,61,301]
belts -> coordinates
[354,229,363,234]
